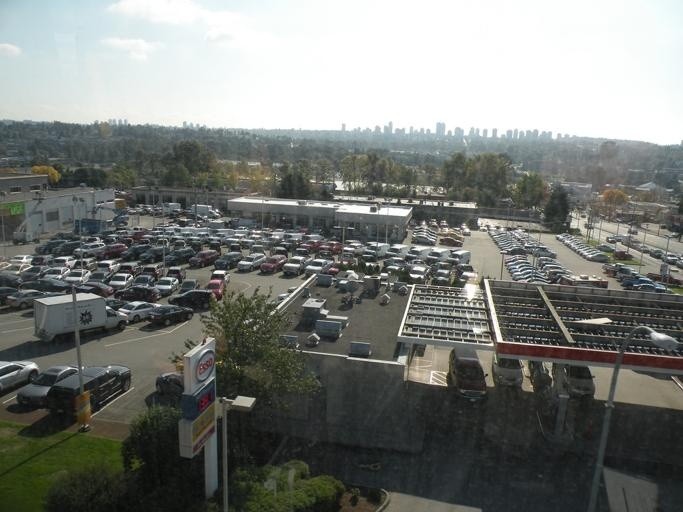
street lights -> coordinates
[587,326,677,512]
[219,395,257,512]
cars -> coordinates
[488,229,568,283]
[0,203,288,326]
[555,233,610,262]
[596,234,683,294]
[493,352,524,387]
[282,219,473,285]
[0,360,40,394]
[564,365,595,399]
[17,366,84,406]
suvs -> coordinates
[47,365,132,417]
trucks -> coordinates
[33,293,129,342]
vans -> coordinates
[449,347,488,399]
